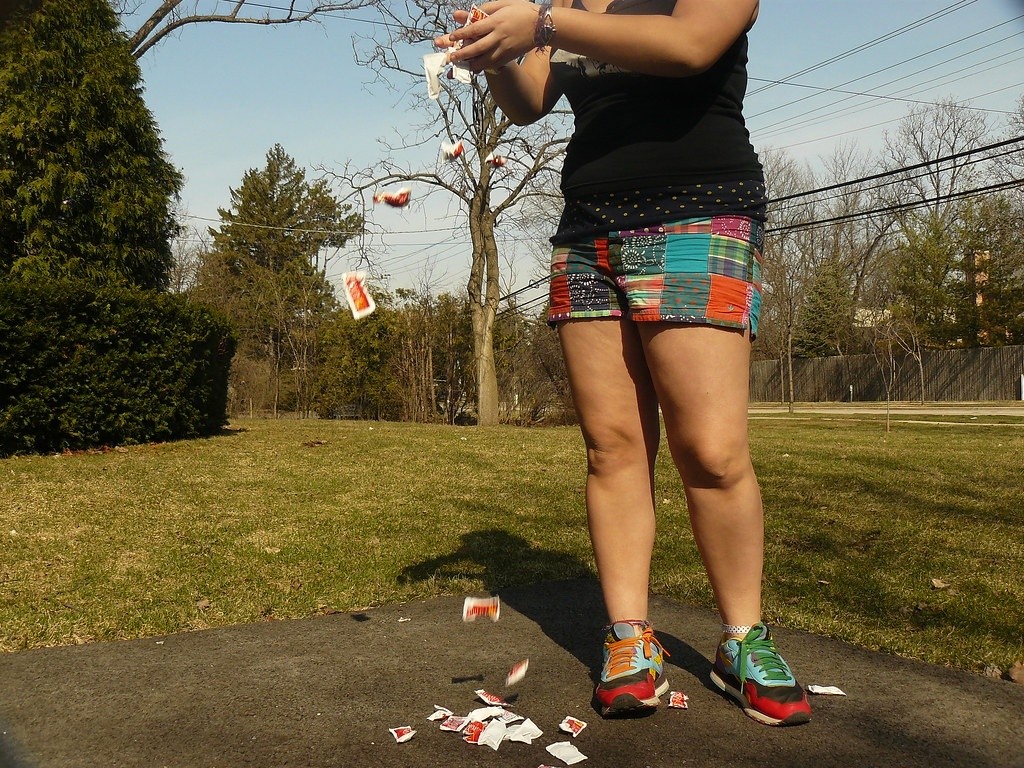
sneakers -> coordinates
[594,621,670,717]
[709,620,813,725]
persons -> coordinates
[435,0,810,725]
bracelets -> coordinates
[533,1,556,51]
